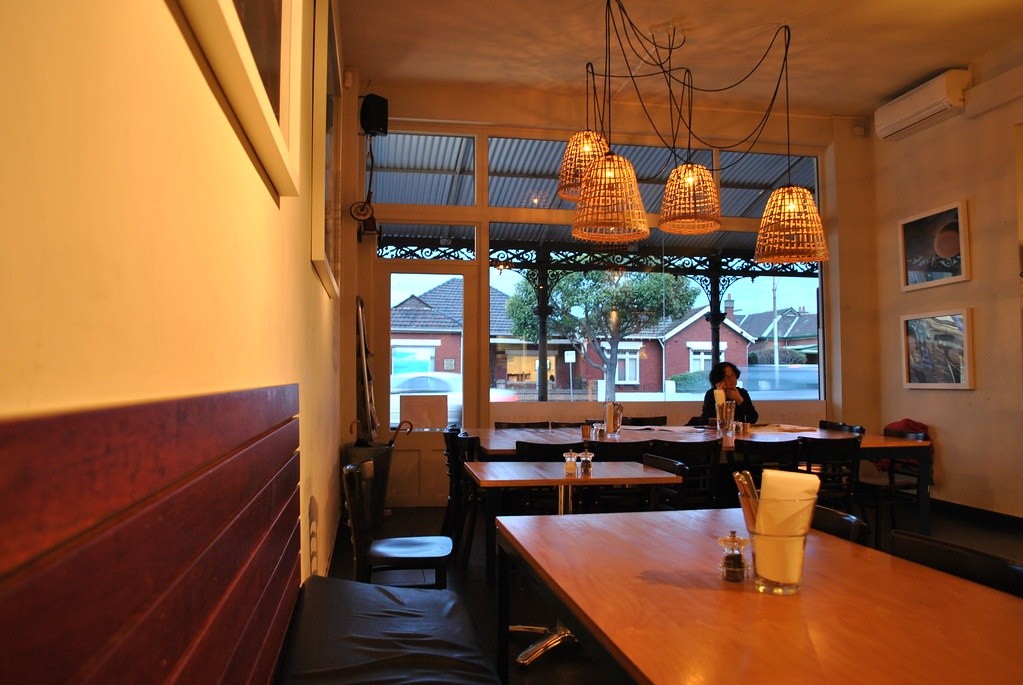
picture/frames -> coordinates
[896,199,972,293]
[897,305,975,389]
[177,0,305,199]
[311,0,343,301]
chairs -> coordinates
[889,528,1023,598]
[441,416,943,586]
[343,463,454,590]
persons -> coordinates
[701,362,759,424]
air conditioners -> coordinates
[874,68,969,144]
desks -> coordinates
[494,506,1023,685]
[456,424,933,541]
[461,459,683,676]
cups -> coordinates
[602,406,623,437]
[715,404,735,434]
[580,426,592,437]
[709,418,717,427]
[738,489,817,596]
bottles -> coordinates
[563,449,595,475]
[734,420,742,433]
[593,423,605,433]
[719,530,750,584]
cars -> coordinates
[390,372,518,426]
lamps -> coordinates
[750,184,830,264]
[554,127,610,205]
[655,162,721,236]
[571,151,649,246]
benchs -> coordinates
[0,382,503,685]
